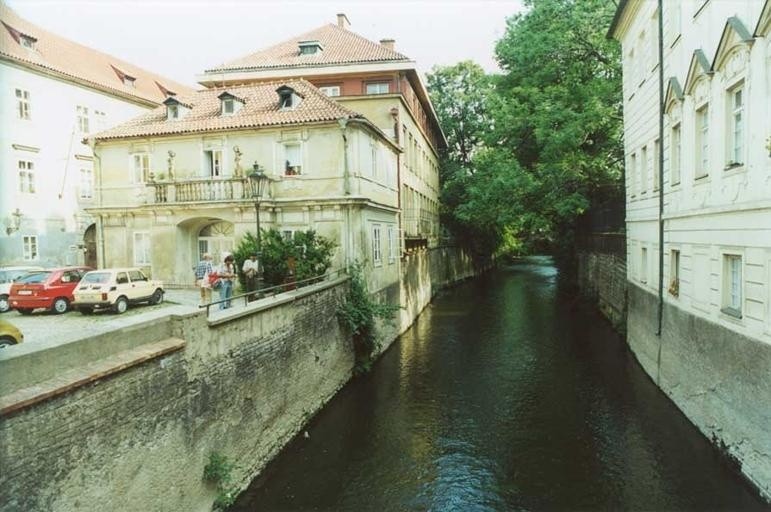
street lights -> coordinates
[249,171,267,296]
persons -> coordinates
[195,252,215,305]
[217,254,239,310]
[242,252,266,303]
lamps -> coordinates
[7,208,23,235]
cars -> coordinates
[0,321,23,351]
[0,265,44,313]
[8,266,96,314]
[71,267,164,315]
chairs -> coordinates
[120,277,128,283]
